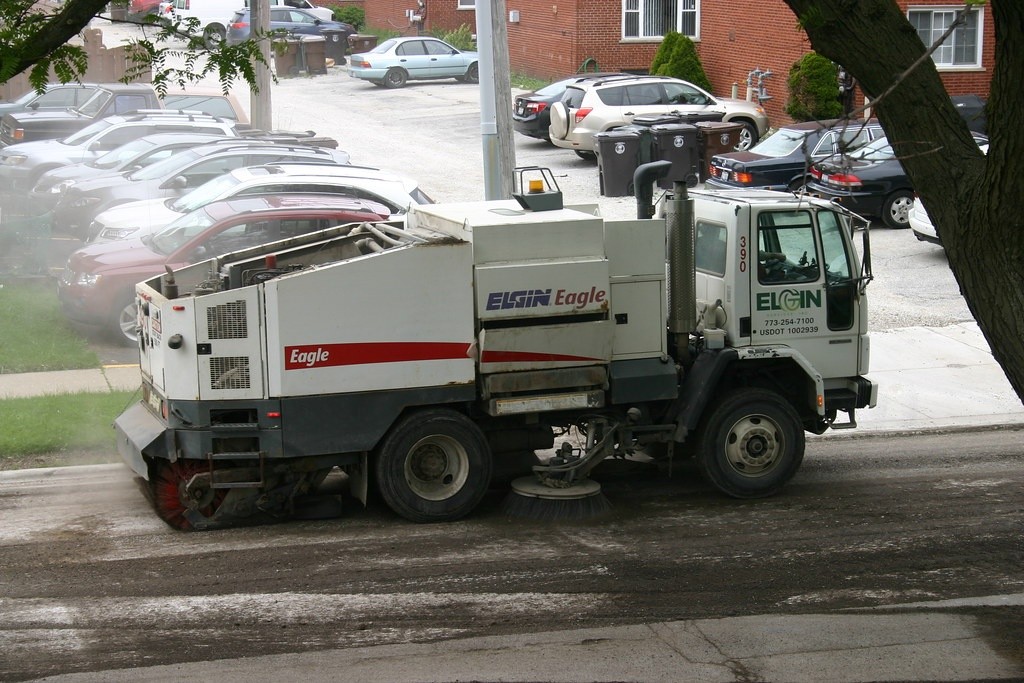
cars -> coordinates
[344,35,480,89]
[703,115,884,198]
[907,142,990,247]
[0,80,432,348]
[512,71,634,143]
[224,4,356,62]
[803,127,991,230]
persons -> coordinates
[837,66,856,113]
[698,222,786,263]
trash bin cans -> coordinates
[695,121,746,183]
[592,125,651,197]
[300,35,327,75]
[631,110,726,125]
[347,34,379,54]
[650,124,697,189]
[319,28,347,66]
[274,33,303,77]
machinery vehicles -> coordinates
[108,156,882,534]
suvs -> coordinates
[547,73,772,160]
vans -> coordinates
[155,0,336,51]
